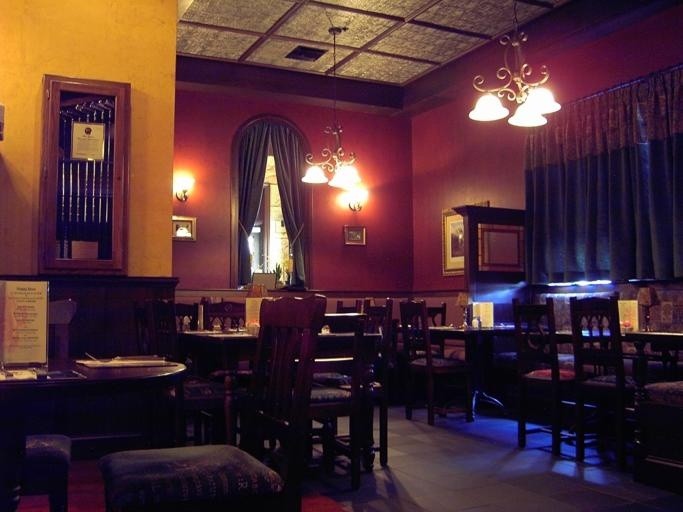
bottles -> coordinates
[471,316,481,328]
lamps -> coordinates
[297,24,368,195]
[467,0,564,130]
[168,170,197,204]
[332,185,367,217]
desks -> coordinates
[428,325,551,421]
[5,358,187,436]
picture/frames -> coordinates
[440,199,490,277]
[343,224,367,246]
[171,214,197,243]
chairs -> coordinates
[100,294,327,512]
[136,294,446,414]
[511,296,682,487]
[399,299,465,424]
[1,386,71,512]
[308,297,393,487]
